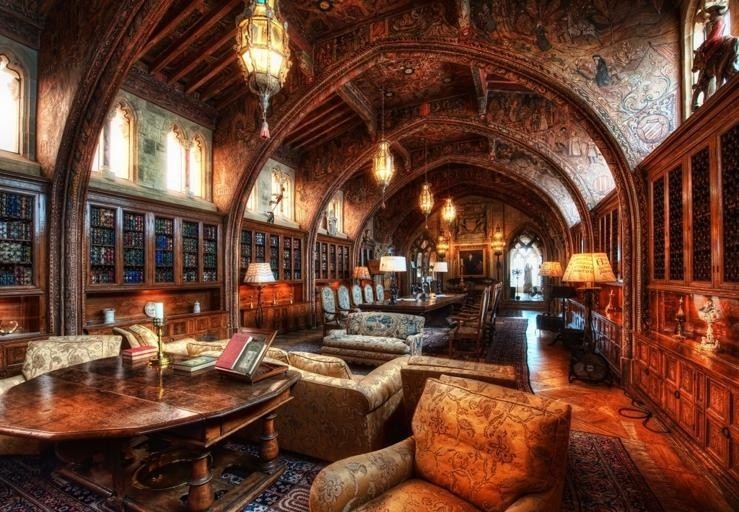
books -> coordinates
[215,332,253,370]
[234,341,268,375]
[123,345,158,356]
[174,355,216,373]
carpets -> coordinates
[0,432,661,511]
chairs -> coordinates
[319,281,504,360]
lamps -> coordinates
[371,62,458,229]
[235,0,292,142]
[378,255,406,305]
[538,252,623,390]
[432,262,448,295]
